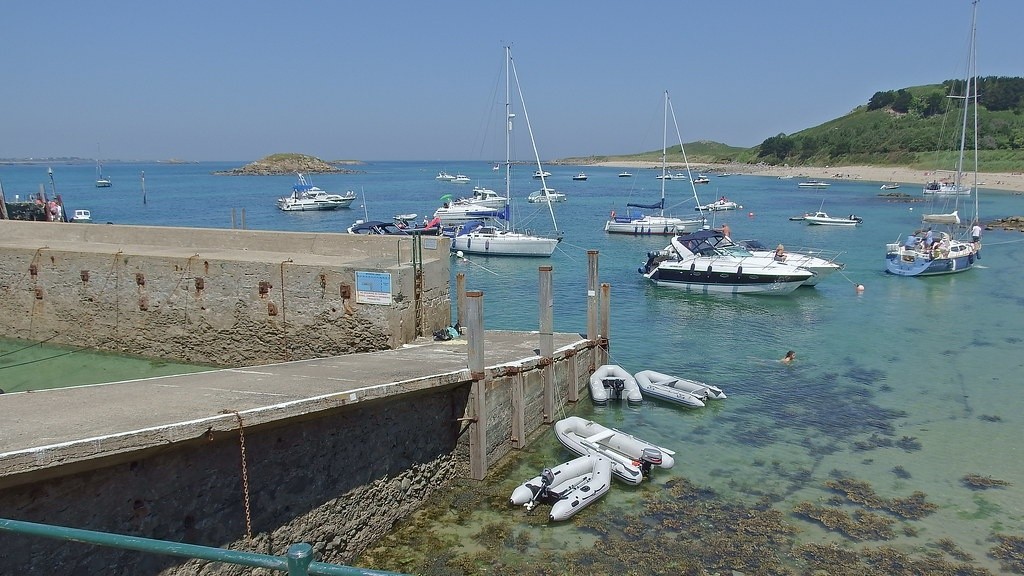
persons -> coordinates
[774,244,787,261]
[905,233,919,251]
[720,196,726,202]
[925,227,933,249]
[717,224,730,237]
[972,222,982,242]
[783,350,795,363]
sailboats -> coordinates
[885,0,982,277]
[94,140,115,188]
[443,46,561,258]
[603,91,711,234]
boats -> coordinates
[634,369,727,408]
[638,232,813,295]
[451,174,471,184]
[277,172,356,208]
[589,365,643,403]
[619,171,632,177]
[435,170,456,181]
[802,212,863,227]
[282,192,345,210]
[922,178,972,195]
[433,199,500,224]
[347,216,443,236]
[798,180,831,189]
[70,208,95,223]
[655,172,731,184]
[533,169,552,178]
[880,183,900,190]
[553,416,674,486]
[573,172,590,180]
[456,186,513,208]
[392,213,418,220]
[528,186,566,203]
[695,201,738,211]
[734,238,841,286]
[509,454,611,522]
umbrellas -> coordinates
[440,194,451,199]
